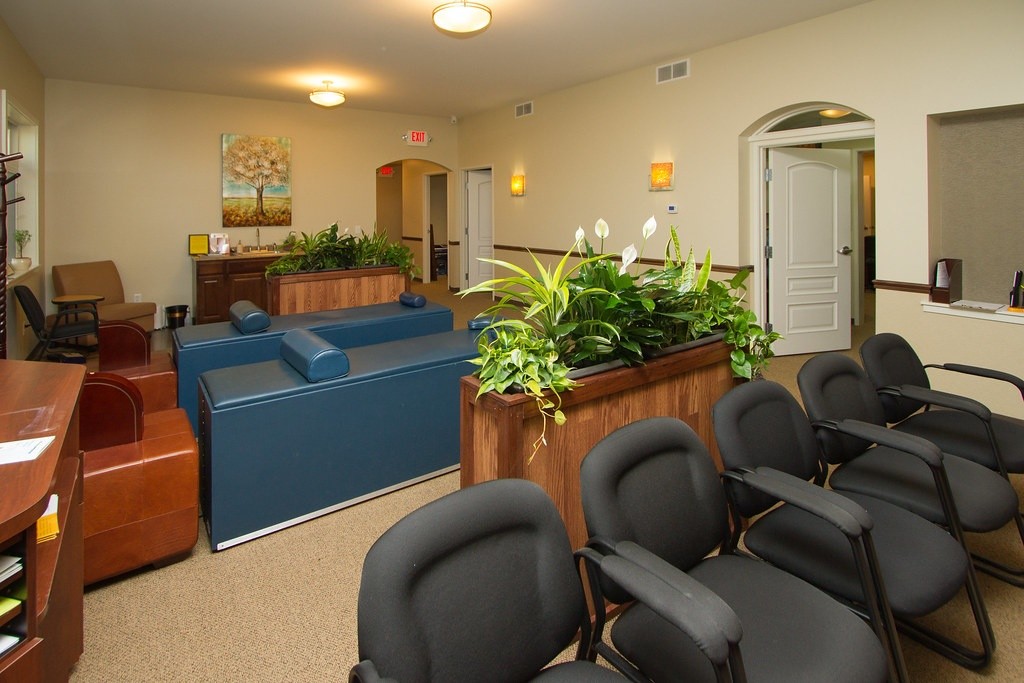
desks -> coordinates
[51,295,105,349]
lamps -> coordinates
[510,176,525,196]
[431,0,492,33]
[648,162,674,191]
[376,166,396,177]
[310,81,345,107]
[402,130,432,146]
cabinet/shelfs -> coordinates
[191,250,306,324]
[0,361,86,683]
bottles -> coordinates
[184,307,192,325]
[237,240,243,253]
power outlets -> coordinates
[355,225,361,233]
[134,294,141,302]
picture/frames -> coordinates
[189,234,209,256]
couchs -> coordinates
[78,321,199,587]
[52,260,156,352]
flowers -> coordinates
[454,216,787,466]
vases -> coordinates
[458,330,749,645]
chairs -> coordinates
[14,285,99,360]
[348,332,1024,683]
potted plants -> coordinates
[265,221,421,316]
[12,230,32,271]
